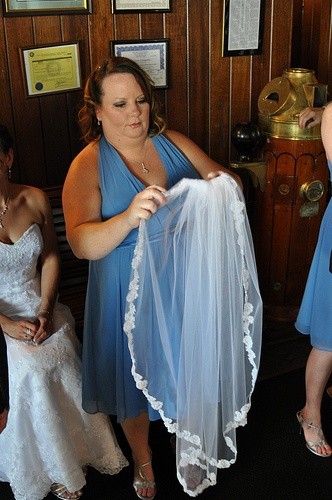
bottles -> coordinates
[232,121,260,163]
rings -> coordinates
[25,327,34,334]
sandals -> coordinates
[49,484,80,500]
[131,447,156,499]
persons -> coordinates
[0,133,132,500]
[60,57,244,500]
[293,101,332,457]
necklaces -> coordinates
[121,146,157,175]
[0,193,14,231]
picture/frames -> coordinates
[3,0,94,17]
[110,0,173,14]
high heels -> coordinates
[296,408,332,458]
